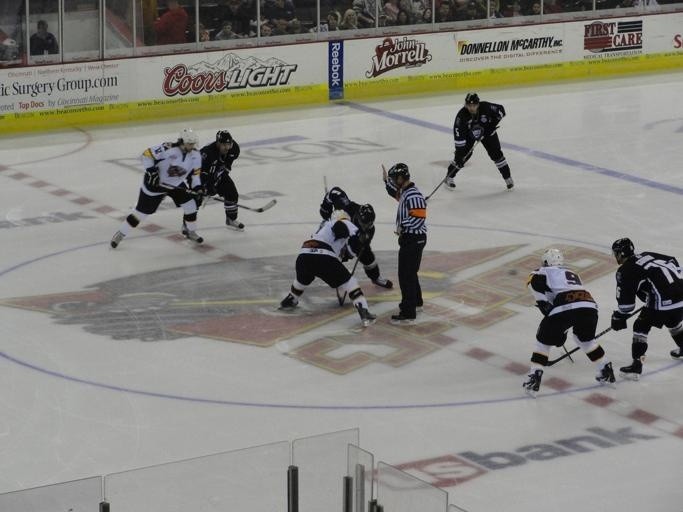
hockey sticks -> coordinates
[545,304,644,365]
[558,341,575,362]
[423,137,483,200]
[157,181,278,213]
[335,244,366,304]
[323,175,342,304]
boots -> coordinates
[390,311,417,326]
[504,177,514,190]
[370,272,393,288]
[277,292,299,311]
[181,219,204,246]
[594,361,616,384]
[108,230,125,249]
[398,299,424,312]
[522,369,543,399]
[619,358,643,381]
[225,214,245,232]
[443,174,456,192]
[669,346,682,359]
[354,303,377,327]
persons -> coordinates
[611,238,683,380]
[182,129,244,243]
[30,19,59,54]
[445,93,513,190]
[320,187,392,289]
[110,129,203,249]
[277,208,377,328]
[154,1,189,44]
[521,248,617,398]
[192,0,682,41]
[381,163,427,325]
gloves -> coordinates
[360,233,372,247]
[556,330,567,346]
[191,187,209,198]
[205,182,217,196]
[215,168,229,185]
[143,168,160,192]
[610,310,627,331]
[319,201,332,220]
[484,118,498,136]
[455,149,468,167]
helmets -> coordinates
[178,127,199,145]
[330,209,351,223]
[611,238,635,259]
[357,203,375,233]
[464,92,479,105]
[215,130,232,144]
[540,247,563,267]
[387,163,410,179]
